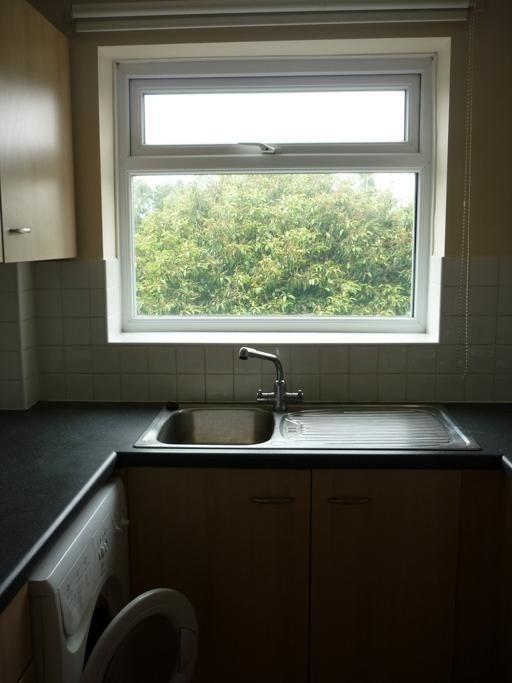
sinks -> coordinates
[132,397,279,447]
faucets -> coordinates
[237,347,307,411]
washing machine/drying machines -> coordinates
[29,475,202,683]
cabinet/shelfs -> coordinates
[0,5,79,264]
[132,469,466,683]
